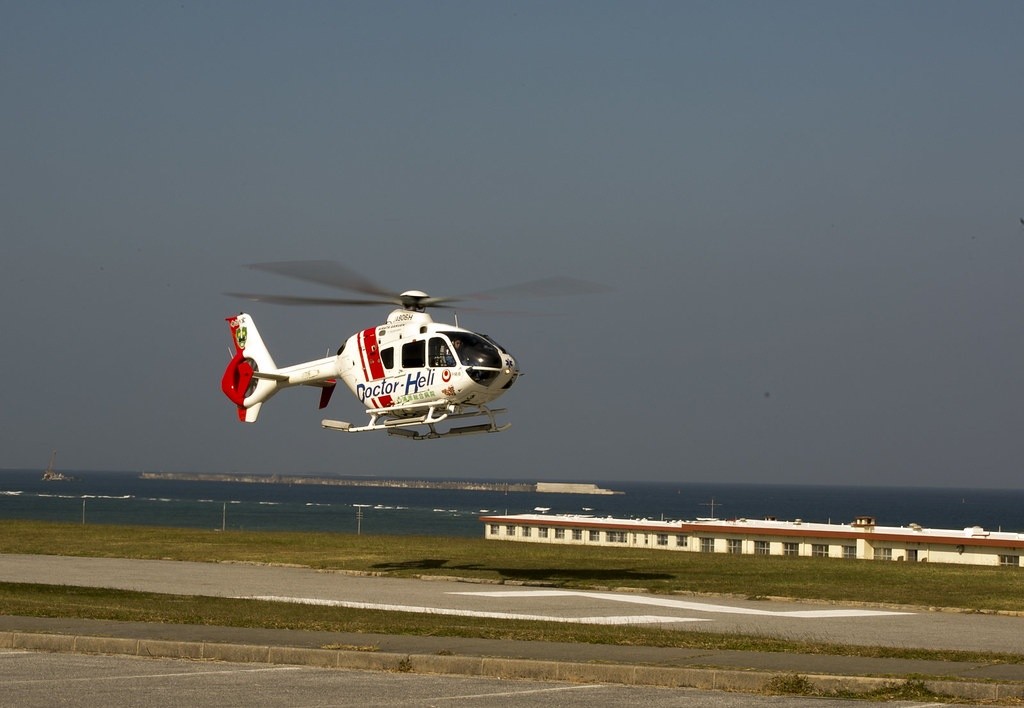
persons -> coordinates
[444,338,465,367]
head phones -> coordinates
[452,341,463,347]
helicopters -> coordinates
[220,254,617,439]
